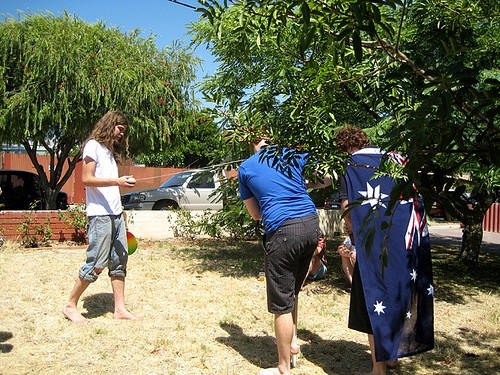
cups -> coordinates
[259,273,266,282]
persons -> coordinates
[238,127,328,375]
[62,110,138,324]
[335,126,435,375]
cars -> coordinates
[118,169,241,210]
[0,168,68,210]
